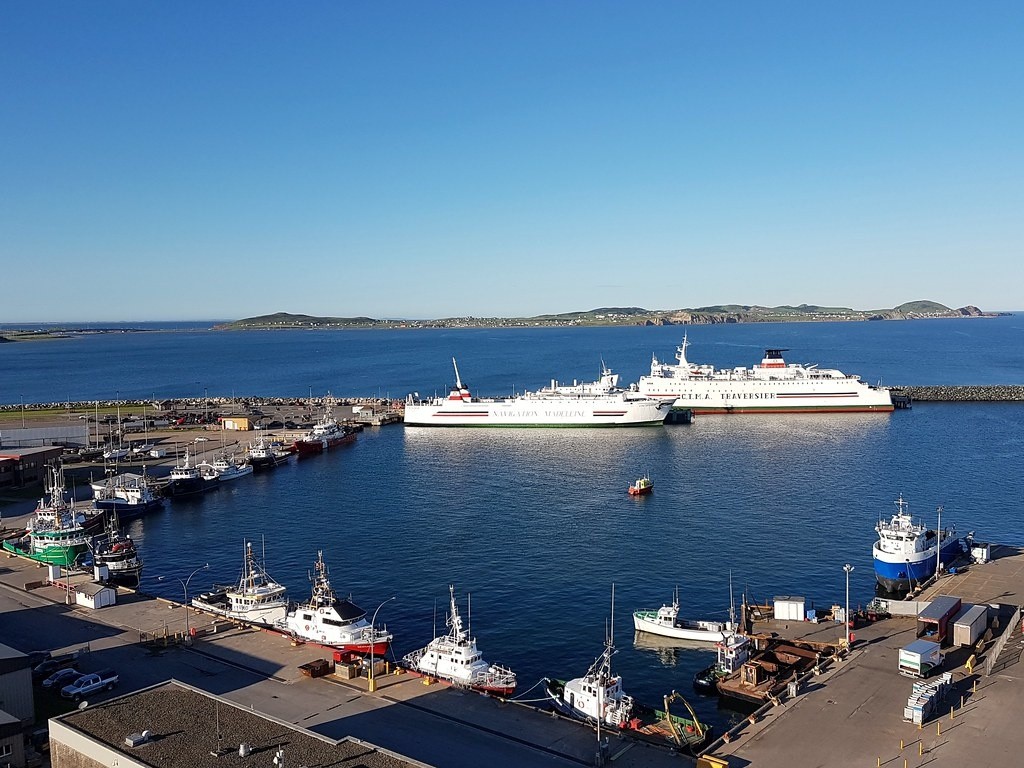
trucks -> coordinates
[897,639,946,680]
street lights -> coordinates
[843,562,854,645]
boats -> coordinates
[628,469,655,495]
[190,536,290,629]
[871,492,969,593]
[294,406,357,454]
[166,440,220,495]
[1,457,166,589]
[132,406,155,453]
[78,398,104,457]
[271,550,394,658]
[211,429,253,482]
[102,405,131,458]
[632,584,739,642]
[401,584,518,699]
[245,426,291,471]
[539,581,715,755]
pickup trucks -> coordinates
[59,667,120,702]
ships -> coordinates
[403,357,683,426]
[626,328,895,413]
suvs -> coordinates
[27,649,88,695]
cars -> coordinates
[194,436,210,442]
